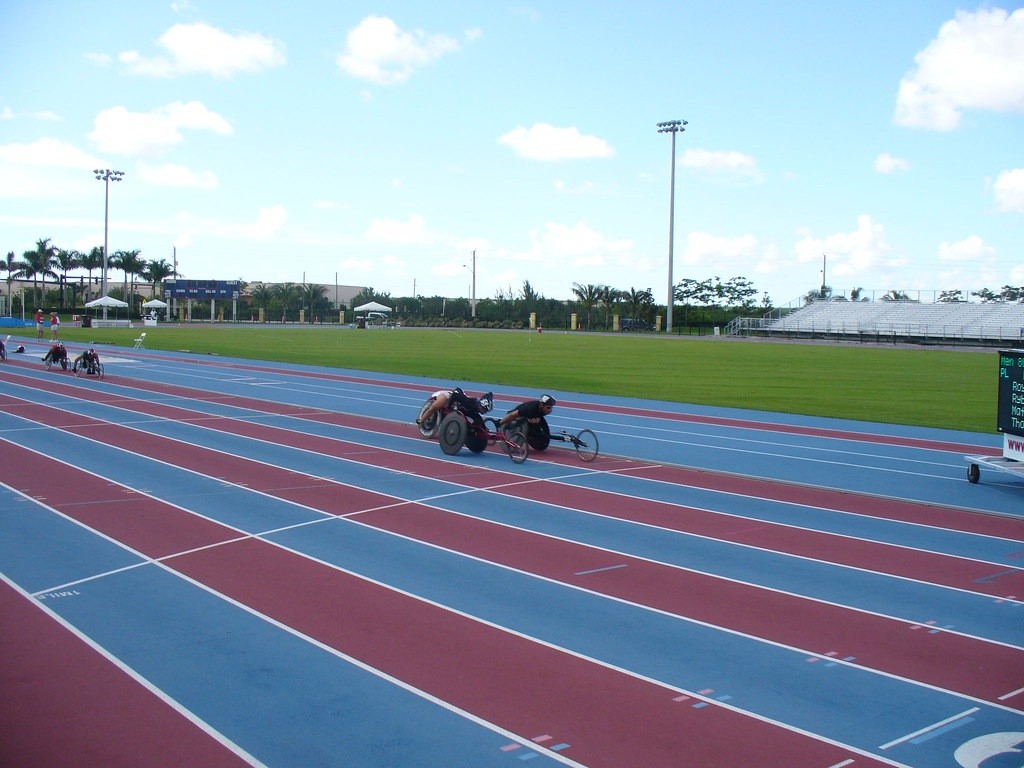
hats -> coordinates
[50,312,55,316]
[37,309,43,313]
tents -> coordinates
[142,299,167,316]
[353,301,392,323]
[85,296,129,320]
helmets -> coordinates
[454,387,467,398]
[479,392,493,412]
[57,342,64,348]
[88,348,95,355]
[540,395,556,405]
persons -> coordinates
[150,309,157,319]
[494,394,556,436]
[417,387,494,433]
[72,348,99,374]
[0,339,5,360]
[35,309,44,342]
[49,312,60,342]
[41,342,67,364]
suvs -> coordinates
[620,318,654,331]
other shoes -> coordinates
[49,339,58,343]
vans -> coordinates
[368,312,389,321]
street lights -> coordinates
[463,249,475,317]
[655,119,688,334]
[93,168,125,320]
